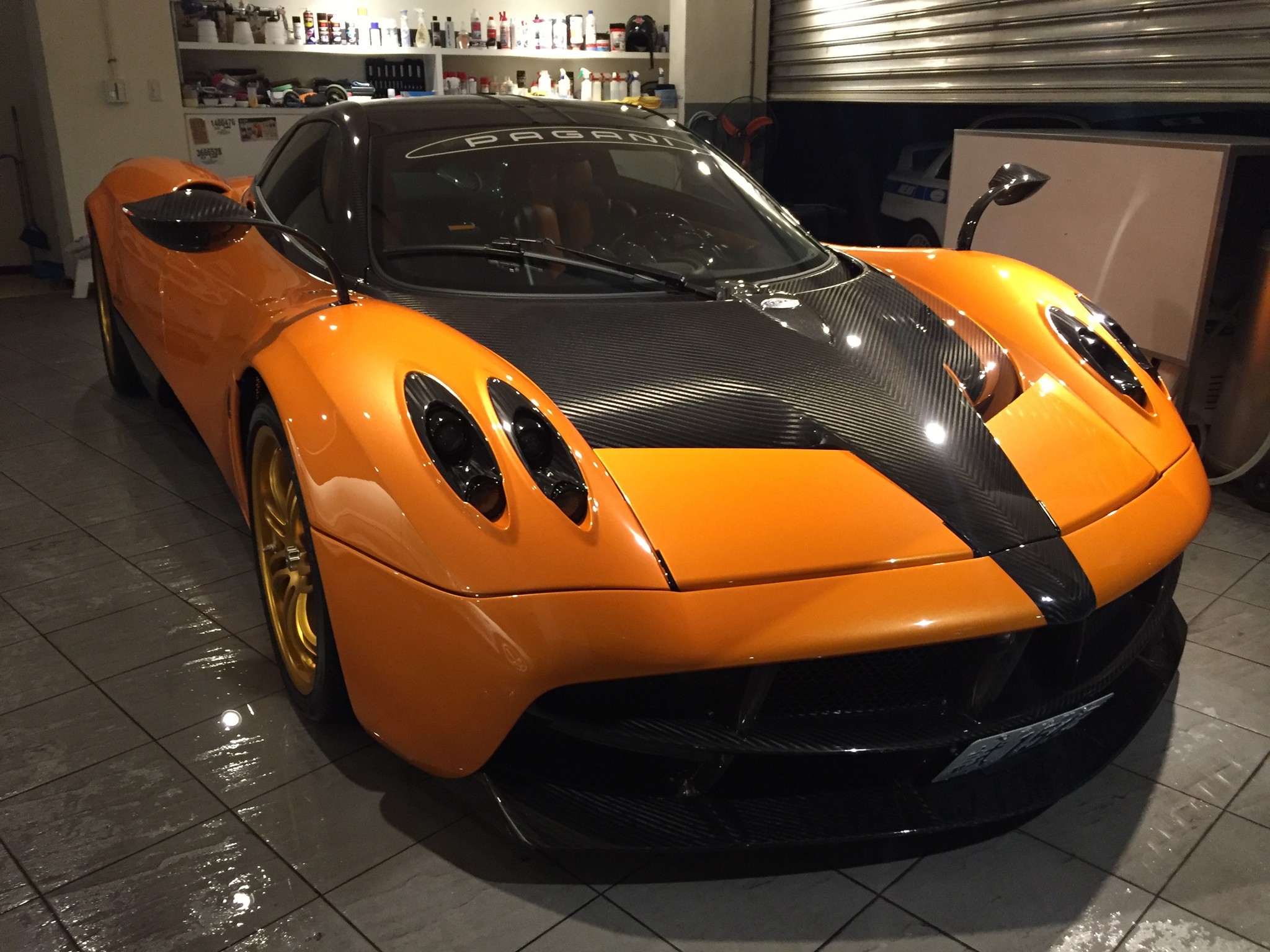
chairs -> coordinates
[517,152,628,280]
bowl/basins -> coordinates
[221,97,236,107]
[236,101,248,107]
[203,99,219,106]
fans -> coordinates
[711,93,781,184]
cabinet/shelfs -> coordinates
[171,0,680,129]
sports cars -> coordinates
[83,97,1214,867]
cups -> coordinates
[183,89,199,108]
[247,86,259,108]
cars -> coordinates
[873,139,974,250]
